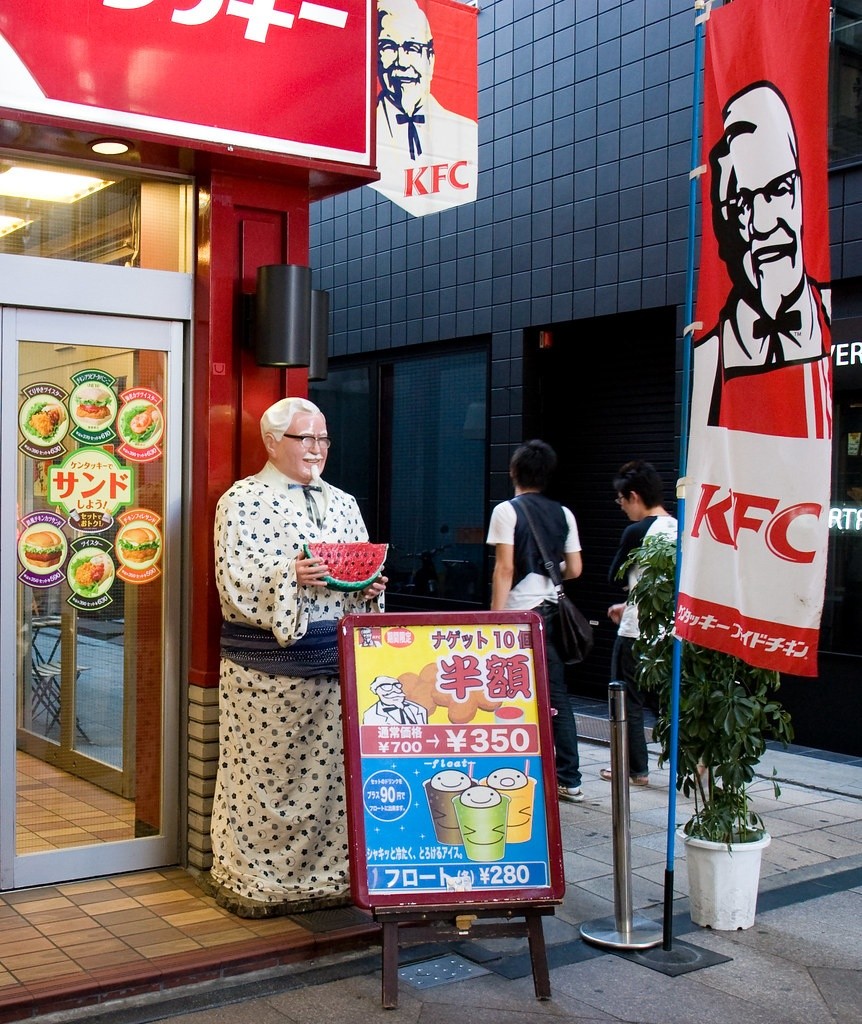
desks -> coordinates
[32,615,80,722]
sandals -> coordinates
[556,784,584,803]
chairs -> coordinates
[32,656,95,747]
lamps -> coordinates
[252,264,311,370]
[303,286,331,387]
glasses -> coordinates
[615,495,625,505]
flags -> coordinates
[672,0,836,680]
[364,1,481,221]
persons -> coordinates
[598,454,707,786]
[484,438,584,808]
[210,396,390,924]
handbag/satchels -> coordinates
[548,595,596,671]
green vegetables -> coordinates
[72,557,98,596]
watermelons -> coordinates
[304,542,387,591]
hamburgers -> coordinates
[120,528,159,562]
[24,532,63,566]
[75,387,112,425]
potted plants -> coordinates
[622,530,798,930]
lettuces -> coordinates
[121,405,155,443]
[25,401,59,443]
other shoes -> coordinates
[599,769,612,782]
[632,775,649,786]
